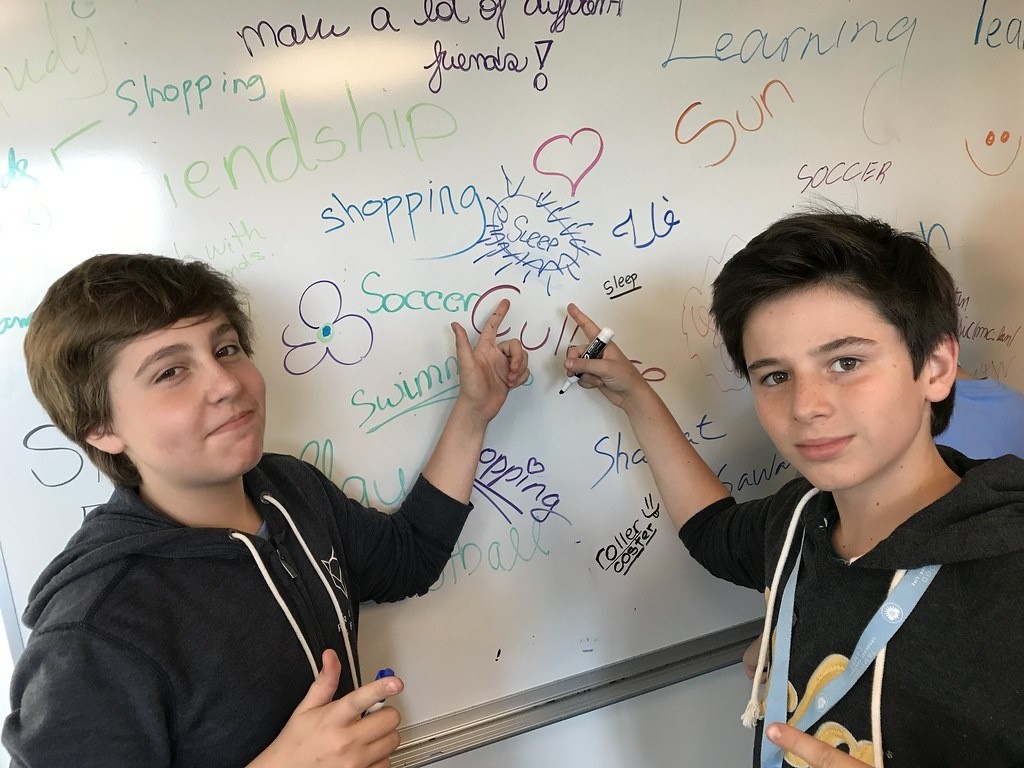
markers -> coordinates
[559,324,615,397]
[361,667,396,717]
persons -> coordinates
[1,254,530,768]
[565,192,1024,768]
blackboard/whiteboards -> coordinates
[0,0,1023,768]
